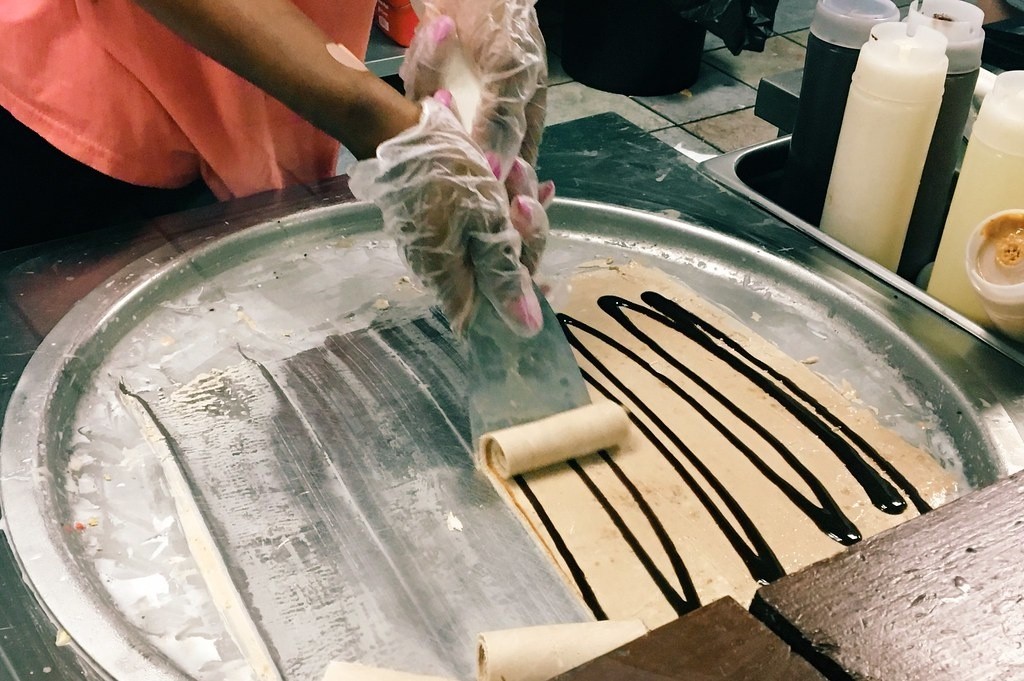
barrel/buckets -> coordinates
[561,1,707,96]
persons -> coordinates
[0,0,555,342]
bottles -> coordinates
[782,0,1024,347]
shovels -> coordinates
[436,90,595,470]
